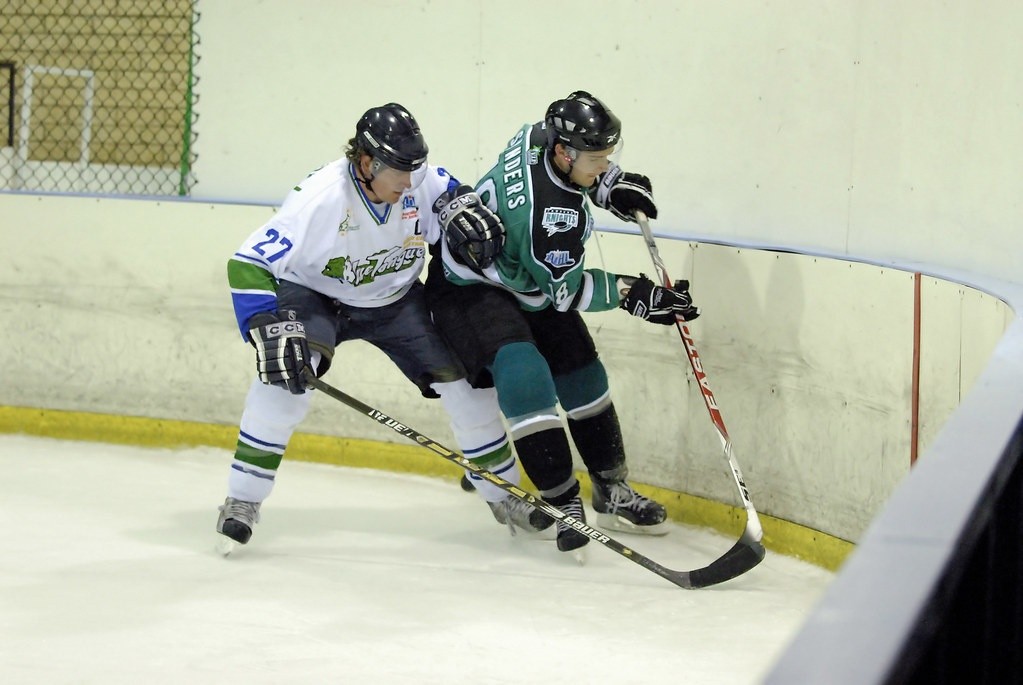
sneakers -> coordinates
[216,496,261,556]
[461,474,558,540]
[589,469,668,534]
[554,492,590,564]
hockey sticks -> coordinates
[304,371,766,591]
[635,208,764,565]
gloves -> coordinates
[432,185,507,269]
[247,303,316,394]
[586,159,658,224]
[620,273,701,326]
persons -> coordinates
[423,91,700,564]
[215,103,557,558]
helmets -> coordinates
[356,103,429,171]
[545,90,621,155]
